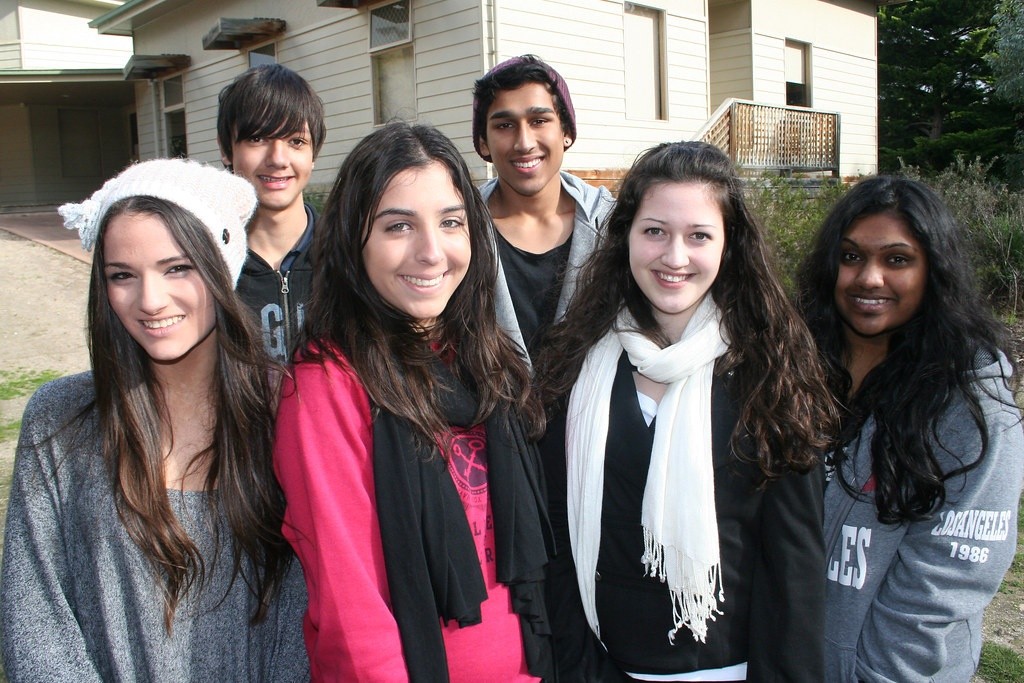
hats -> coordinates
[56,158,257,291]
[472,56,577,163]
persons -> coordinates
[0,155,311,683]
[216,63,326,366]
[470,55,617,378]
[527,141,840,683]
[272,116,559,683]
[791,173,1024,683]
